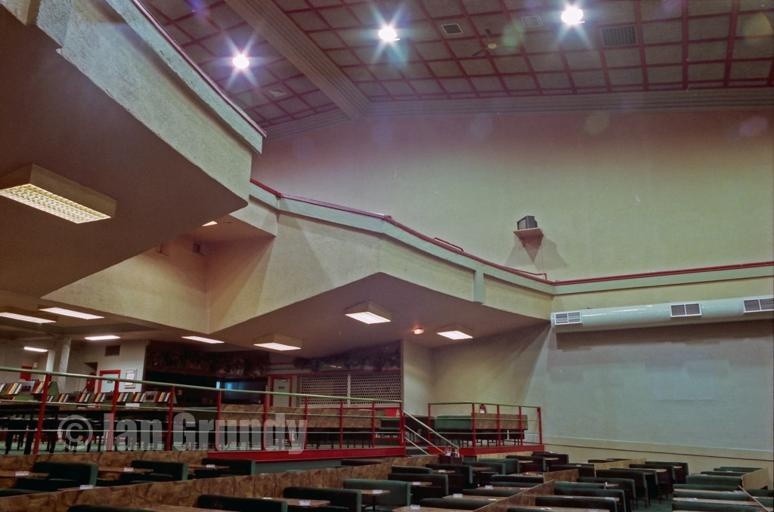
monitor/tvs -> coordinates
[517,216,537,229]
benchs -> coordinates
[0,451,774,511]
[381,414,529,446]
